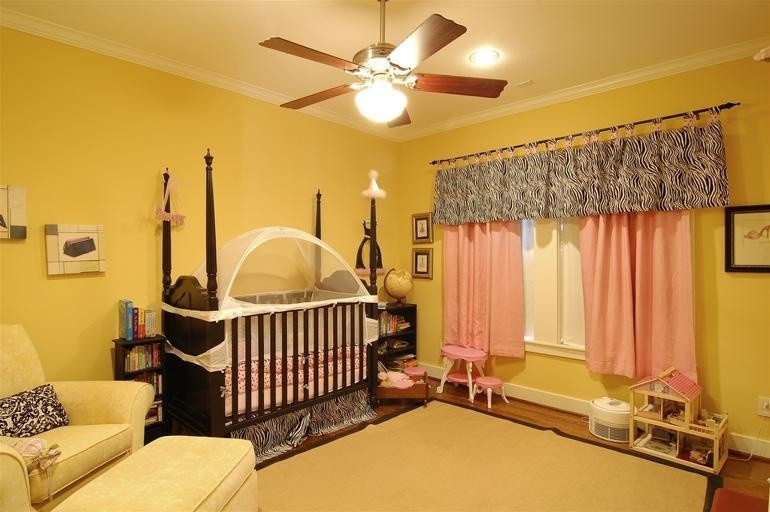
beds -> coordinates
[161,147,386,471]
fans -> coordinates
[248,2,508,128]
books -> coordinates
[118,298,165,427]
[378,309,417,369]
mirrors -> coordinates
[356,220,385,276]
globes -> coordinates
[383,267,414,306]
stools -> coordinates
[46,435,260,512]
[473,377,509,408]
[435,342,488,402]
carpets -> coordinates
[253,399,722,512]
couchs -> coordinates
[0,327,155,511]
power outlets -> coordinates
[758,395,770,421]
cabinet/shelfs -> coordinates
[110,335,169,444]
[376,303,416,372]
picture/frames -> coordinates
[0,184,29,242]
[42,221,107,275]
[409,246,435,278]
[723,204,770,274]
[410,211,435,244]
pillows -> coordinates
[1,382,69,437]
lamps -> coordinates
[351,80,409,128]
